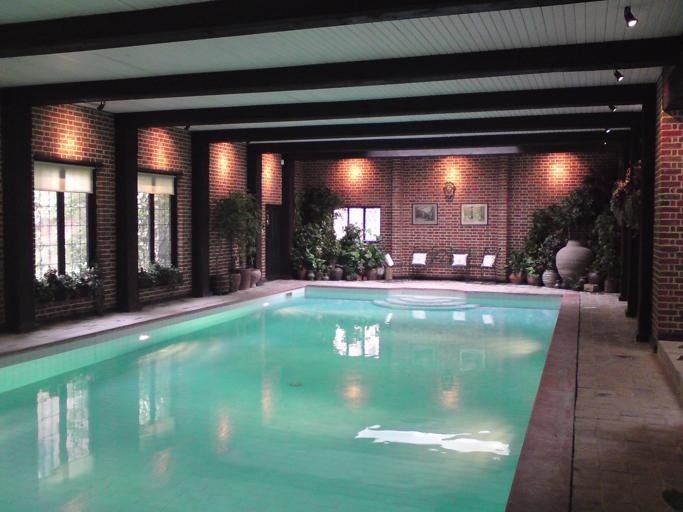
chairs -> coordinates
[406,244,501,285]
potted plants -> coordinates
[217,190,261,290]
[294,221,384,281]
[503,233,618,293]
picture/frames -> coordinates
[459,202,488,227]
[411,203,438,225]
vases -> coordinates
[209,272,241,294]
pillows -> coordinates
[385,252,394,268]
